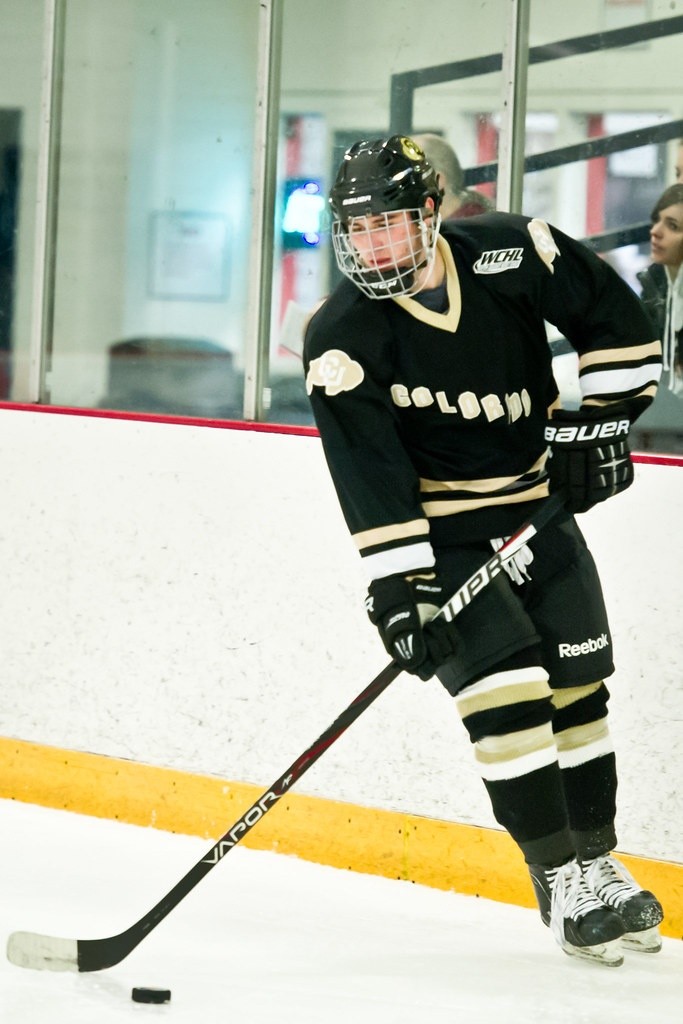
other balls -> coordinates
[130,986,173,1008]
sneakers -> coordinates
[522,859,628,968]
[575,850,663,954]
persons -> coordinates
[611,182,683,455]
[303,136,665,953]
[407,133,496,218]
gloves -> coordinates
[544,404,635,515]
[364,570,463,684]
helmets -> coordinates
[328,136,445,241]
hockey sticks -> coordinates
[3,486,583,976]
